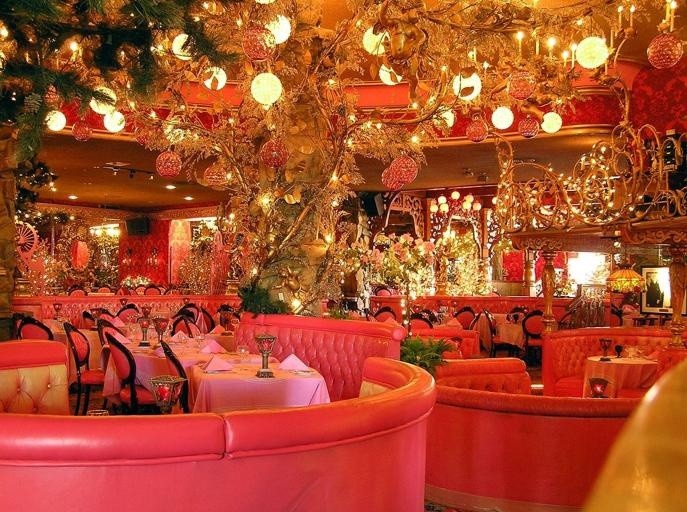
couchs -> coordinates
[425,356,657,511]
[542,326,687,396]
[0,338,437,511]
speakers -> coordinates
[364,192,384,217]
[126,217,150,235]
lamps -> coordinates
[428,196,481,225]
[0,1,687,135]
[606,245,646,293]
[486,72,686,233]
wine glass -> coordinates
[237,343,251,372]
[614,344,646,359]
[128,336,205,357]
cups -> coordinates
[86,410,110,416]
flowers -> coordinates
[364,231,435,285]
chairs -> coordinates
[10,281,330,413]
[326,299,544,367]
